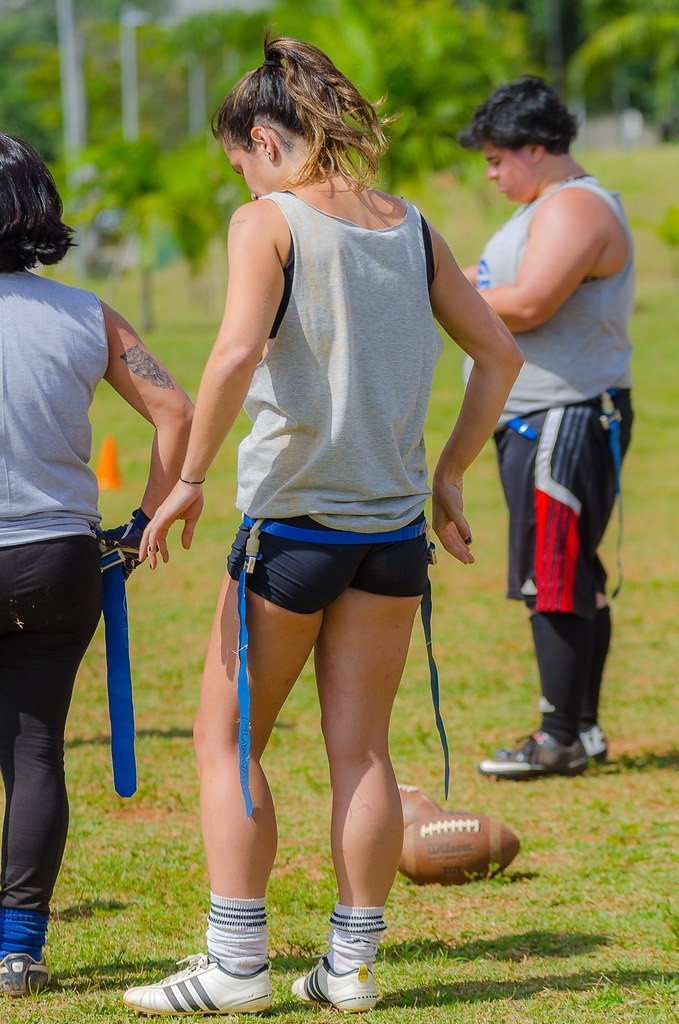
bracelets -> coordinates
[178,474,205,485]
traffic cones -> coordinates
[95,431,125,492]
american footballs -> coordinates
[394,809,523,886]
[397,782,440,816]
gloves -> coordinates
[90,520,159,583]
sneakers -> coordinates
[0,953,51,998]
[479,723,608,777]
[292,954,376,1012]
[123,953,273,1015]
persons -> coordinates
[450,71,637,779]
[0,131,195,999]
[112,31,527,1014]
